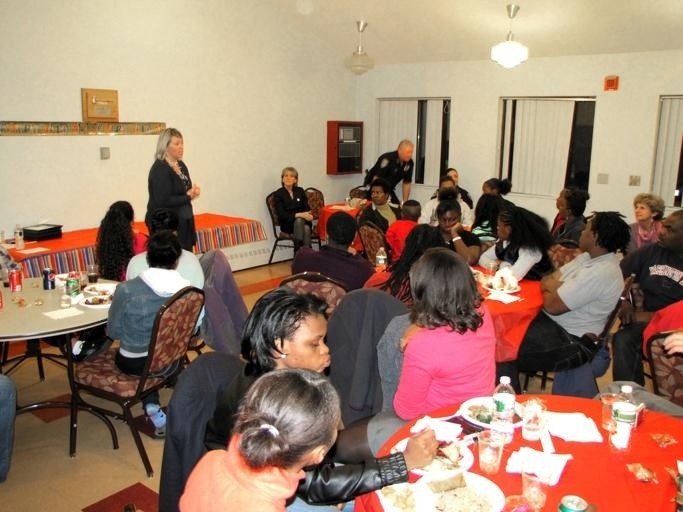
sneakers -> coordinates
[143,402,168,432]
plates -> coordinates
[381,434,508,512]
[472,271,521,296]
[458,395,530,428]
[78,283,121,308]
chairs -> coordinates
[0,184,682,511]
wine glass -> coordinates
[518,467,548,512]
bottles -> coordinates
[674,457,683,511]
[375,245,388,272]
[609,384,637,458]
[342,197,365,207]
[490,375,517,445]
[12,224,23,249]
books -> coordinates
[81,87,119,122]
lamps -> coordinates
[345,20,373,77]
[487,2,532,69]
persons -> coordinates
[143,127,200,253]
[126,205,205,340]
[95,199,148,281]
[1,370,19,484]
[104,230,194,440]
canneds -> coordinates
[9,270,22,292]
[558,495,588,512]
[66,271,81,297]
[43,268,55,290]
[0,290,3,309]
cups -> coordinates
[487,260,510,287]
[477,429,504,474]
[58,287,76,307]
[2,259,11,287]
[600,392,621,431]
[78,263,99,288]
[522,402,548,441]
